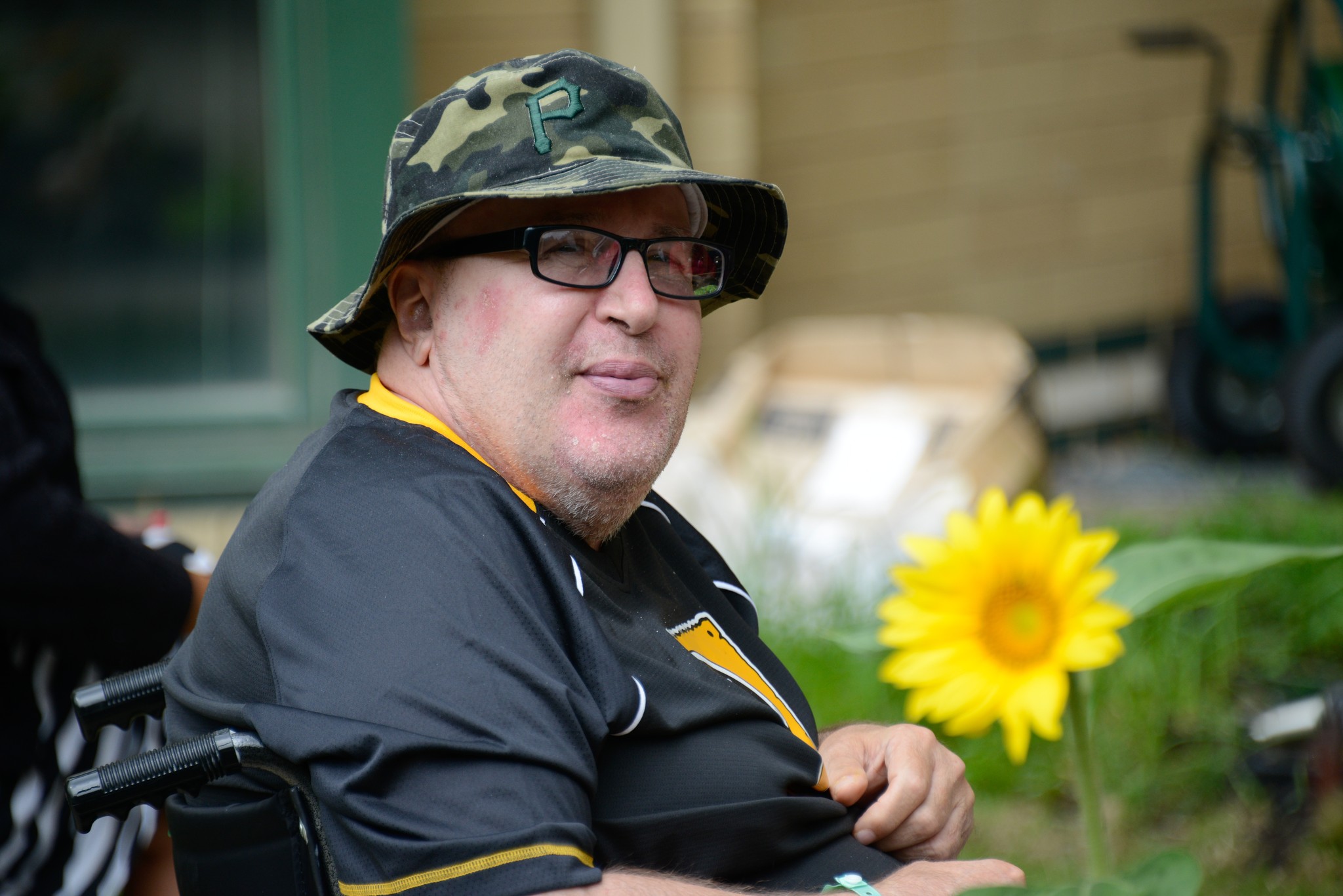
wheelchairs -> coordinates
[59,653,344,896]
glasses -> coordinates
[414,224,726,299]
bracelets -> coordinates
[821,874,882,896]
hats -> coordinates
[305,48,789,375]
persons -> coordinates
[0,288,213,896]
[164,46,1025,896]
